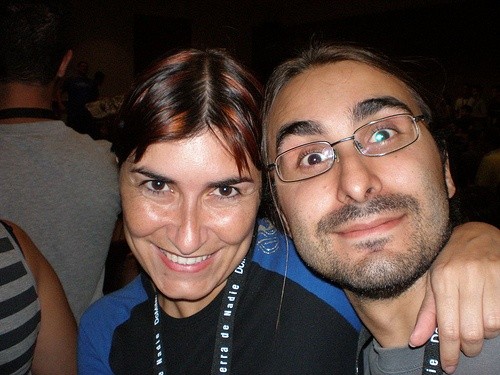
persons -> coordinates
[259,46,500,375]
[421,69,500,215]
[1,0,125,332]
[76,48,500,375]
[0,218,83,375]
[55,50,109,142]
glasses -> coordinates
[265,113,426,182]
